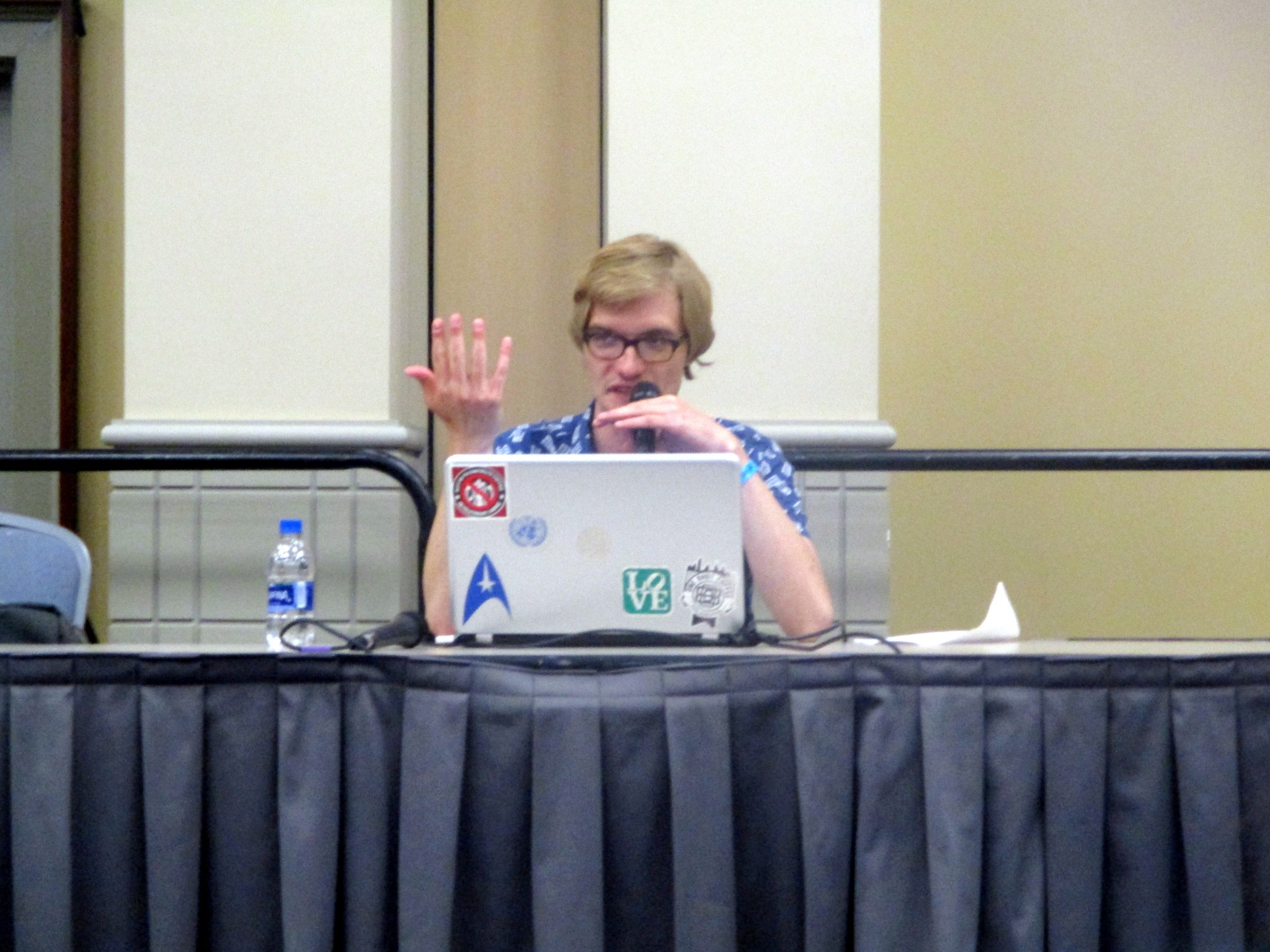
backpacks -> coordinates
[0,600,89,645]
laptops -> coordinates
[444,452,745,646]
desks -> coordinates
[0,638,1270,952]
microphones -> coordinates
[348,610,424,651]
[631,380,660,453]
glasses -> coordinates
[582,328,690,364]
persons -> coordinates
[405,234,834,653]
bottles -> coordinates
[265,519,316,651]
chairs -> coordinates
[0,511,94,633]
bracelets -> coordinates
[737,460,759,484]
